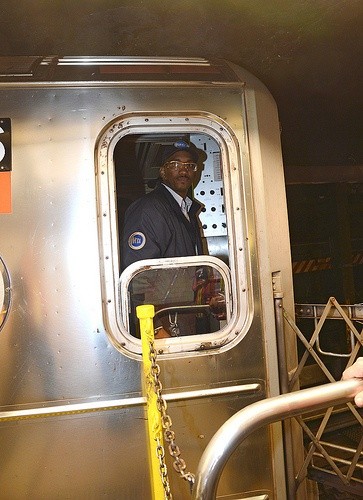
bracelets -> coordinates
[153,326,166,336]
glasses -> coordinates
[165,161,197,172]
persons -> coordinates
[120,139,224,339]
[342,357,363,407]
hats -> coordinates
[168,139,196,157]
